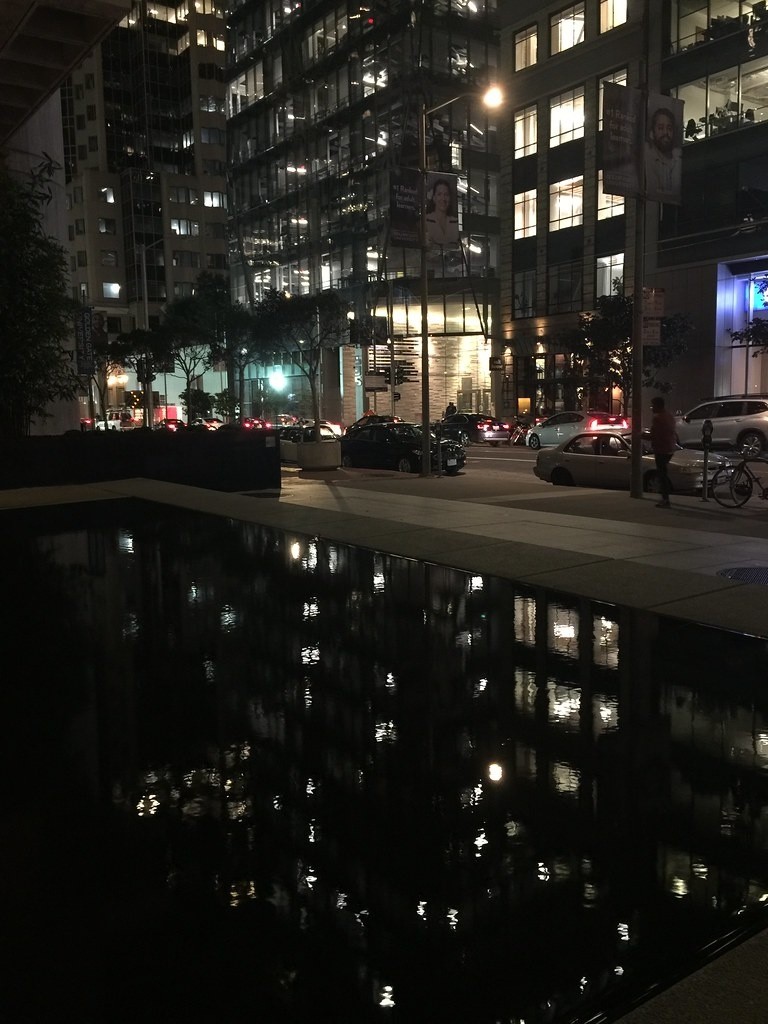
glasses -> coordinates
[649,404,654,408]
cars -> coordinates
[524,409,630,451]
[334,422,467,474]
[673,396,768,461]
[532,426,731,497]
[341,415,405,436]
[80,407,341,443]
[433,412,510,448]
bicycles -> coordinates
[708,445,768,512]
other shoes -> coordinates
[655,499,671,508]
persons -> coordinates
[643,397,678,508]
[646,108,682,192]
[426,180,458,244]
[446,402,456,416]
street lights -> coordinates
[416,88,503,476]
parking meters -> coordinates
[700,419,713,501]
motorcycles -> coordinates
[507,415,531,446]
